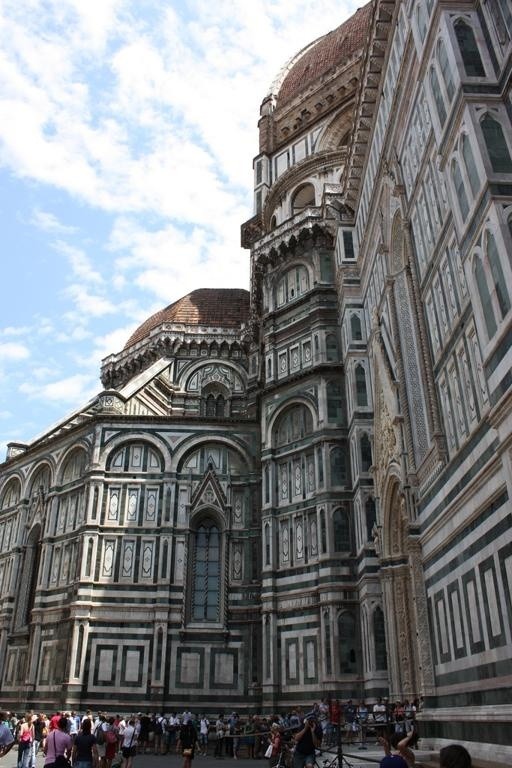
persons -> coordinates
[439,744,472,768]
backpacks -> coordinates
[96,723,106,745]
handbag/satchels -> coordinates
[54,754,72,768]
[182,749,193,756]
[20,731,33,743]
[264,742,274,758]
